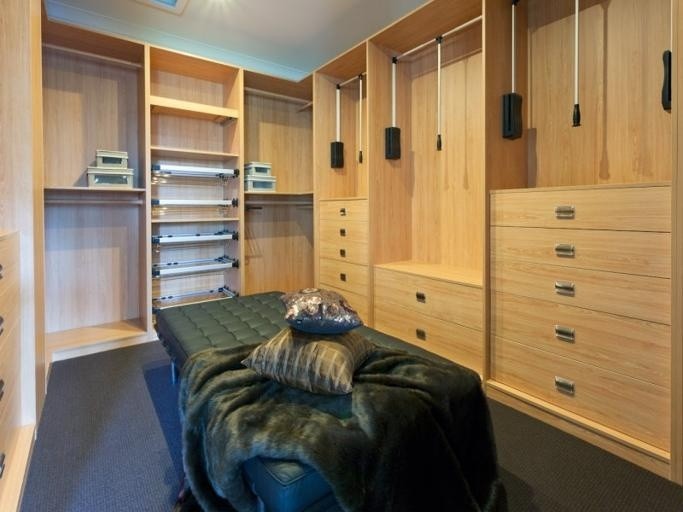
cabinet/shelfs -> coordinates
[312,0,683,470]
[1,3,313,509]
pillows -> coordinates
[236,284,379,396]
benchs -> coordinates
[153,287,480,512]
